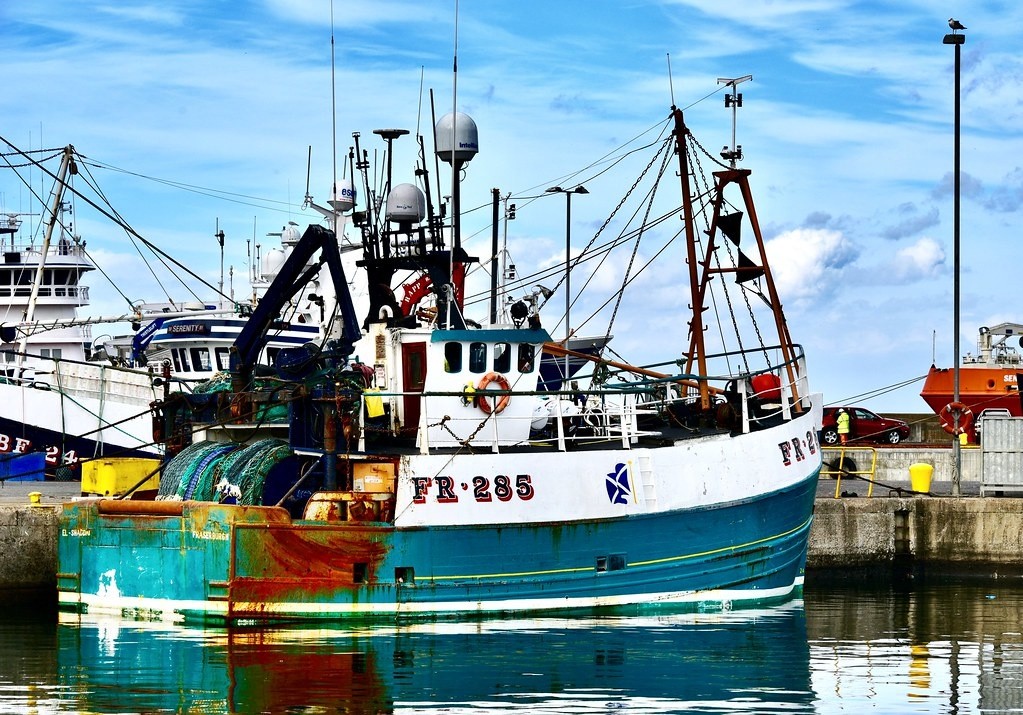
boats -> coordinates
[0,1,841,640]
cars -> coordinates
[819,406,912,446]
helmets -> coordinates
[838,409,844,412]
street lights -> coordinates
[941,17,965,491]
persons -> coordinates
[836,408,850,446]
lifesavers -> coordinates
[939,402,974,436]
[475,372,510,416]
[828,456,858,481]
